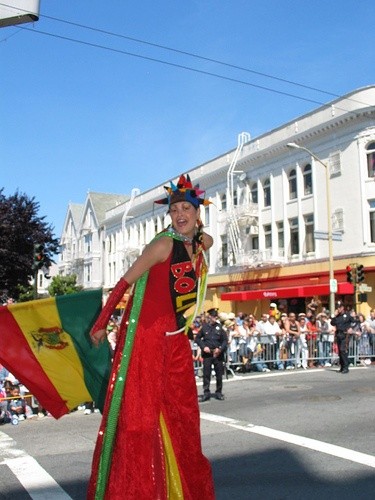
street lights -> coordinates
[286,142,336,318]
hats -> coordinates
[207,308,219,316]
[154,173,213,208]
[229,313,236,319]
[281,313,287,316]
[270,303,276,307]
[336,301,344,307]
[224,320,234,327]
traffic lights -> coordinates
[33,243,44,269]
[345,263,356,284]
[358,293,368,303]
[355,263,365,283]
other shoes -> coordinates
[349,363,353,366]
[94,408,100,413]
[297,364,307,368]
[286,366,294,370]
[199,396,210,402]
[84,409,91,414]
[13,414,25,421]
[219,396,224,400]
[336,370,348,373]
[325,363,331,366]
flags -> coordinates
[0,288,115,419]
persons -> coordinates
[87,173,215,500]
[0,300,375,427]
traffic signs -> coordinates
[359,284,373,293]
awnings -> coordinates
[220,280,354,302]
